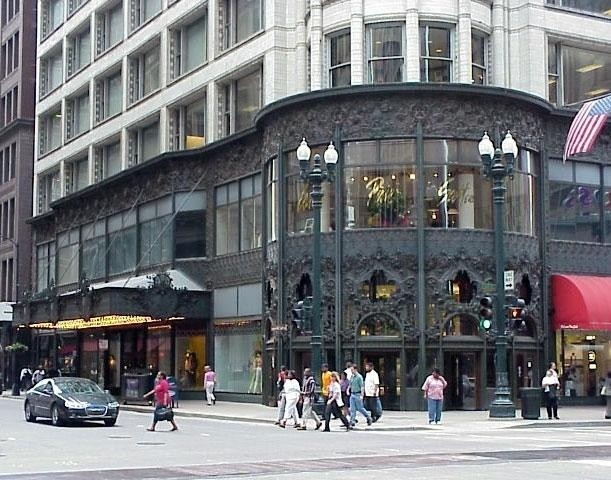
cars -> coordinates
[22,376,121,428]
[464,376,475,396]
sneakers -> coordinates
[430,420,441,425]
[275,416,383,433]
[548,416,559,420]
[147,427,155,432]
[207,399,217,406]
[171,428,177,431]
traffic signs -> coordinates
[503,270,514,291]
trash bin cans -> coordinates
[120,374,152,406]
[519,389,542,419]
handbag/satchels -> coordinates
[154,407,174,422]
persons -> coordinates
[20,358,61,392]
[247,355,257,393]
[109,355,116,387]
[602,372,611,418]
[185,349,198,388]
[143,372,177,432]
[548,362,560,408]
[204,366,217,405]
[253,352,263,394]
[542,370,559,419]
[422,368,448,425]
[274,361,381,431]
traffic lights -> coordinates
[513,298,525,331]
[504,305,522,332]
[478,292,497,335]
[289,297,312,337]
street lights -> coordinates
[294,132,338,415]
[475,126,520,420]
